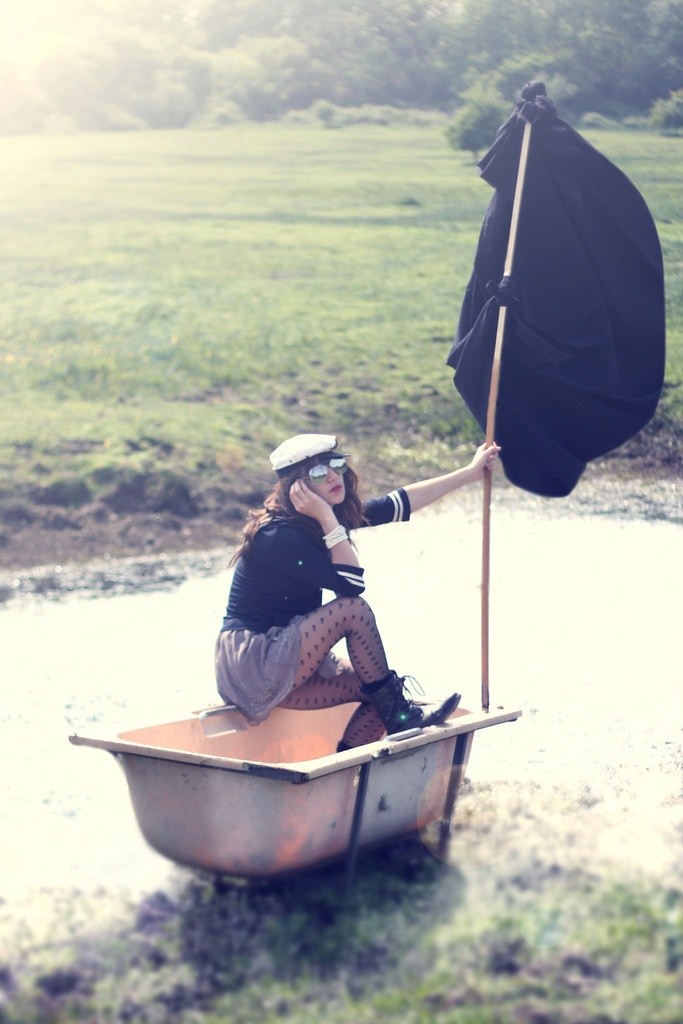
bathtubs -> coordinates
[66,683,523,878]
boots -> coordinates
[359,670,461,736]
[336,741,353,753]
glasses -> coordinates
[302,457,348,483]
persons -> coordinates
[214,432,501,755]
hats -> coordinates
[269,434,351,479]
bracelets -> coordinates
[322,525,349,549]
